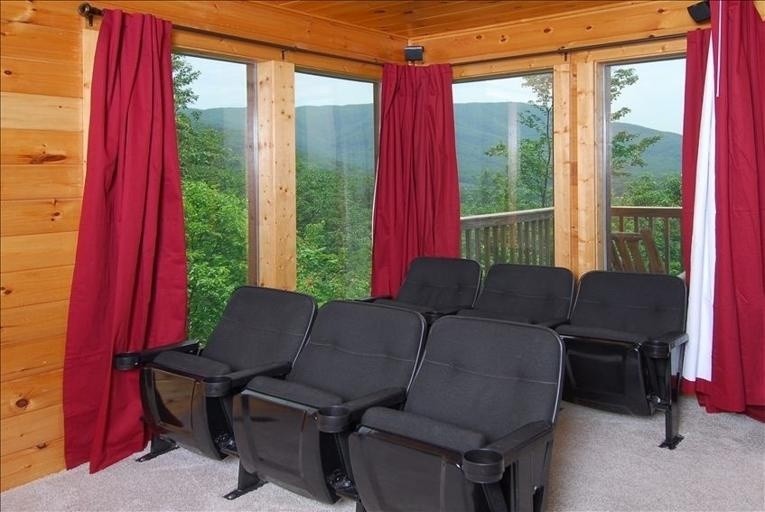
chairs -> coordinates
[115,286,318,510]
[354,256,483,326]
[349,316,566,509]
[458,264,574,337]
[232,297,426,510]
[556,270,690,451]
